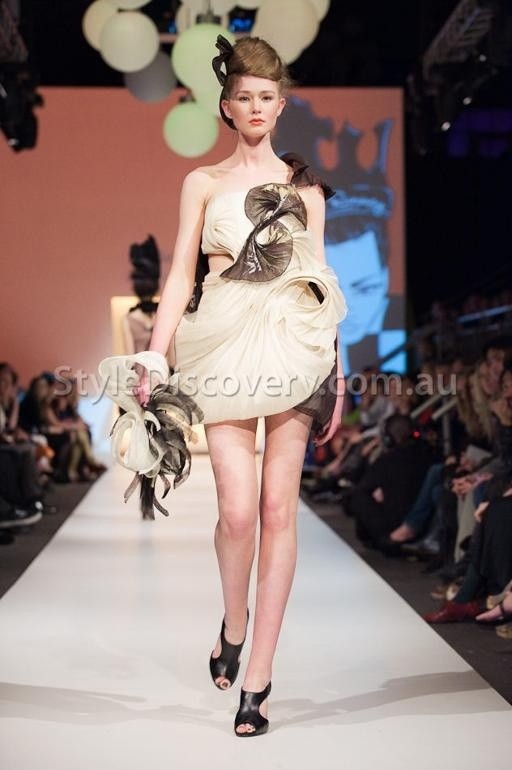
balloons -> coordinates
[81,1,330,156]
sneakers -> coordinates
[0,505,42,528]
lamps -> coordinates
[81,2,332,161]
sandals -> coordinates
[210,608,249,690]
[235,680,271,737]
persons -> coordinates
[300,289,510,627]
[1,359,108,530]
[272,93,407,376]
[121,234,174,519]
[98,37,345,736]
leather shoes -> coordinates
[424,599,480,623]
